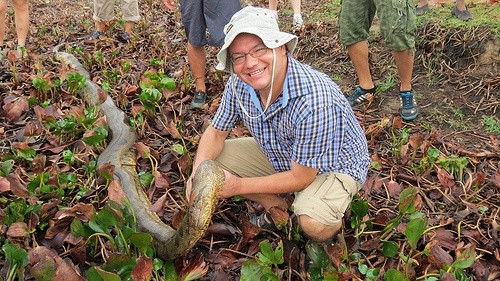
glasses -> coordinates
[231,46,268,64]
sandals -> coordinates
[248,213,281,238]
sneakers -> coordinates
[346,86,380,109]
[84,31,107,44]
[190,90,207,109]
[399,90,419,119]
[17,47,28,58]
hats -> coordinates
[215,6,298,73]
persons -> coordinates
[339,0,419,121]
[80,0,141,46]
[181,0,242,109]
[414,0,473,22]
[268,0,305,34]
[186,7,370,243]
[0,0,30,64]
